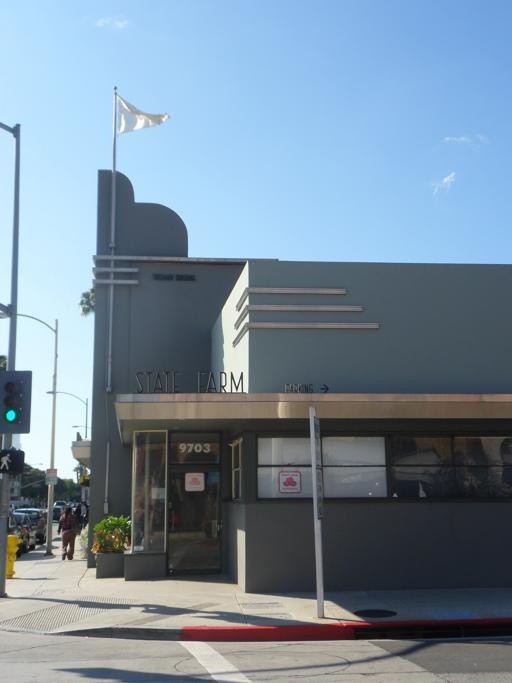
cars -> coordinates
[6,510,31,554]
[13,506,46,551]
[45,499,76,520]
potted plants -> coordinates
[92,517,130,579]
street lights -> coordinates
[45,387,89,439]
[0,303,59,558]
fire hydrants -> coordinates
[6,535,25,579]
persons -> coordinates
[115,93,169,134]
[34,499,88,561]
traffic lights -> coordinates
[0,371,31,433]
[0,447,26,474]
